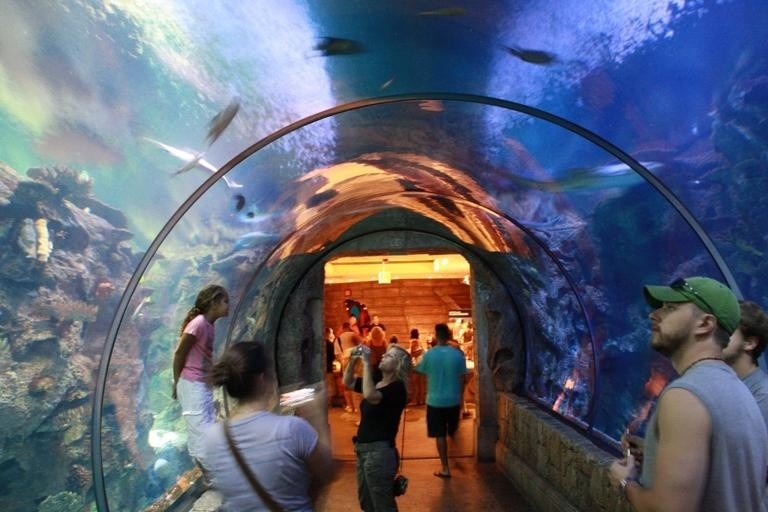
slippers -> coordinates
[433,470,451,478]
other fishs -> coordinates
[63,421,88,445]
[153,458,173,479]
[701,157,758,182]
[311,1,558,114]
[230,231,279,251]
[207,96,241,147]
[498,151,666,194]
[144,136,243,189]
[173,152,203,177]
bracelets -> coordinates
[618,477,640,497]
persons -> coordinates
[722,298,768,426]
[172,285,231,489]
[342,344,413,511]
[190,340,332,511]
[324,314,474,427]
[413,322,467,477]
[609,274,767,511]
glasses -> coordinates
[670,277,714,314]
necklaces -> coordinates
[678,357,726,378]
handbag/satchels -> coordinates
[393,475,408,496]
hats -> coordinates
[643,276,768,357]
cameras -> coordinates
[352,346,363,355]
